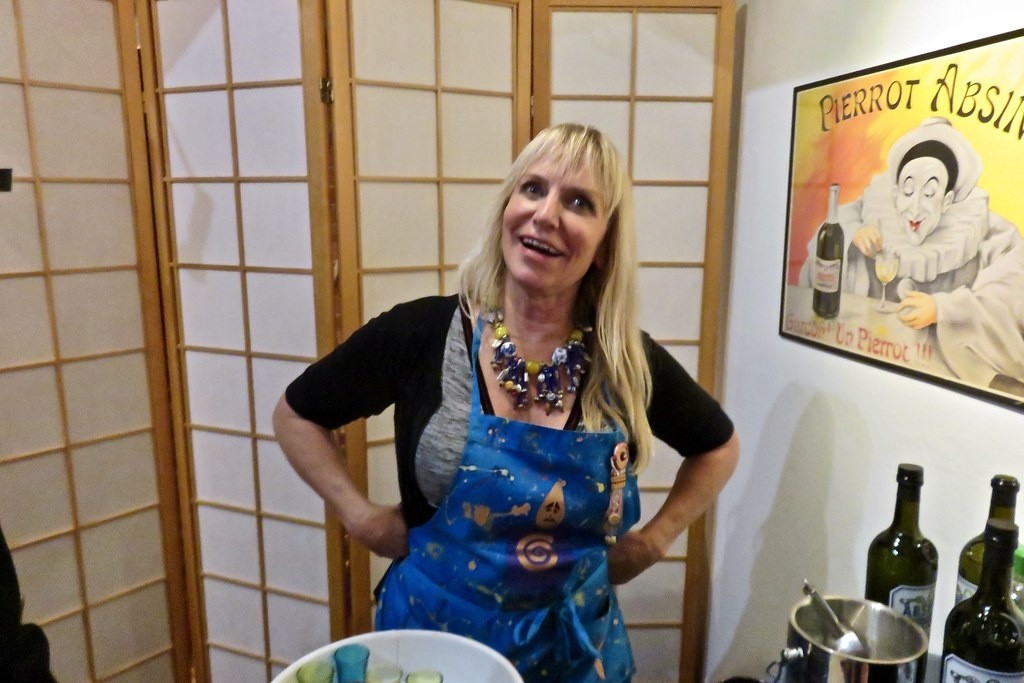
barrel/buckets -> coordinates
[270,629,524,683]
[765,597,927,683]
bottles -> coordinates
[955,475,1021,605]
[939,518,1024,683]
[865,464,938,640]
[297,660,334,683]
[366,665,402,683]
[406,669,443,683]
[335,644,371,683]
[1011,547,1024,612]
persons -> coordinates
[272,121,740,683]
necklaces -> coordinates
[469,288,595,416]
